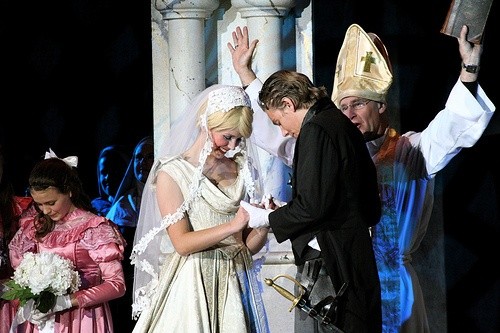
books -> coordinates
[440,0,493,45]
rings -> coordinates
[235,44,239,49]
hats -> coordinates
[331,24,394,110]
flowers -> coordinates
[0,252,83,313]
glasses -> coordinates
[341,99,371,112]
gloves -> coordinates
[261,194,287,210]
[23,294,72,330]
[240,199,275,229]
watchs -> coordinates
[461,62,480,74]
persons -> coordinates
[228,26,496,333]
[239,70,384,333]
[0,148,127,333]
[128,84,288,333]
[88,131,154,255]
